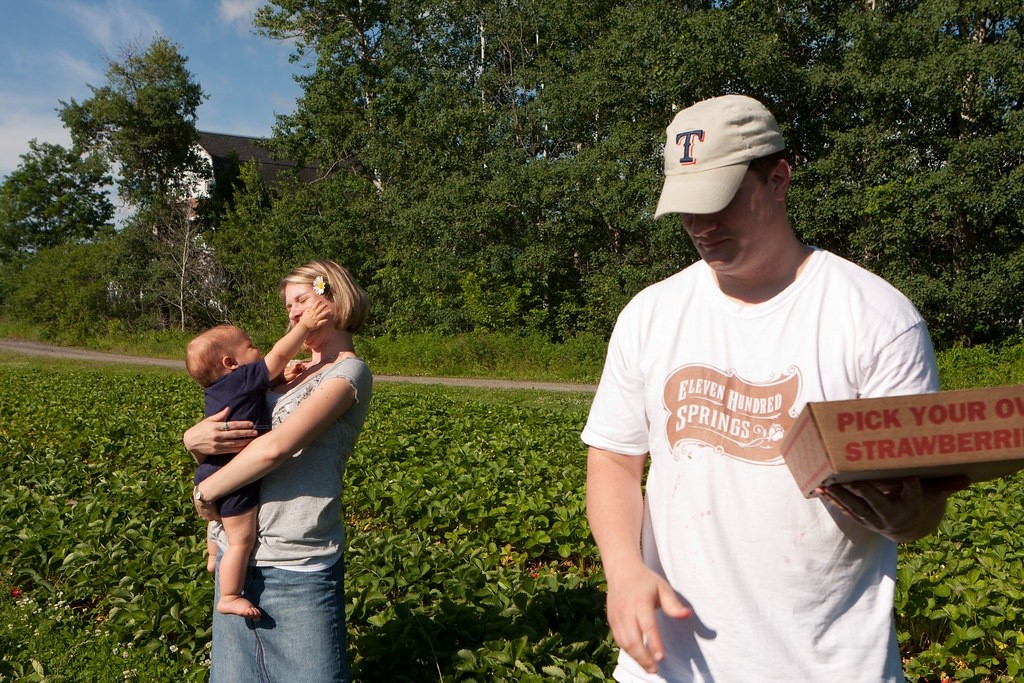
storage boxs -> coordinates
[779,384,1024,500]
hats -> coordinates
[653,94,785,219]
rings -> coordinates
[224,422,229,431]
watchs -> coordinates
[194,486,212,504]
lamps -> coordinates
[314,276,328,296]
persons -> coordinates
[183,261,373,683]
[581,94,972,683]
[186,300,331,618]
